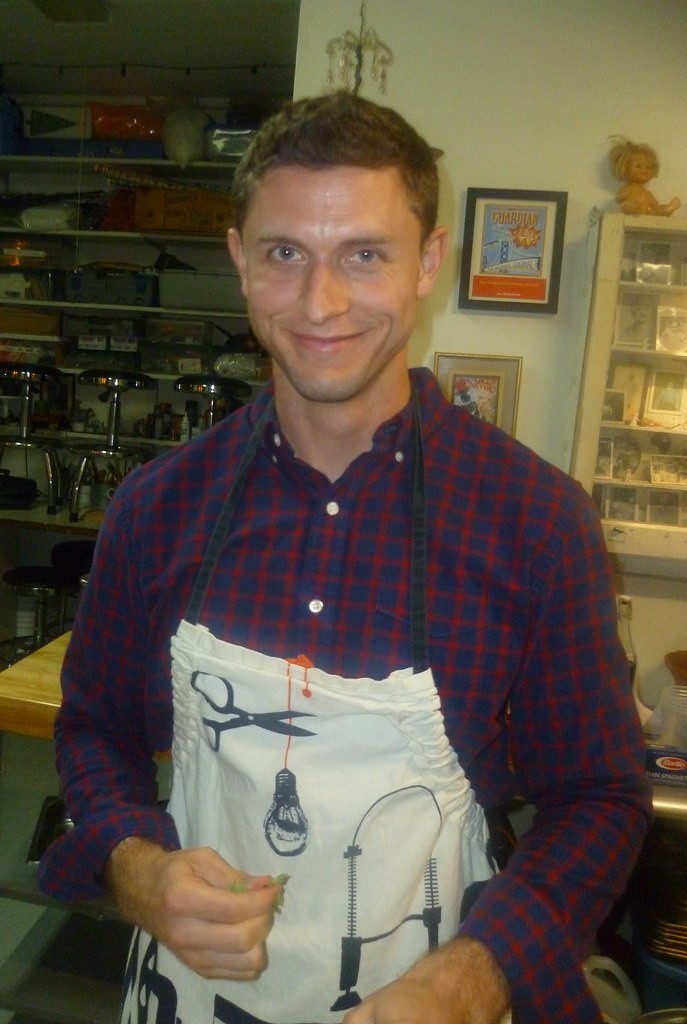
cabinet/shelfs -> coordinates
[0,156,310,645]
[567,211,687,563]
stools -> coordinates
[175,374,253,429]
[68,368,157,523]
[0,537,97,665]
[0,362,63,515]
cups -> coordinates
[637,686,687,747]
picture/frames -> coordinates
[433,353,522,440]
[592,242,687,531]
[459,187,569,312]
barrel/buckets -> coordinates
[627,922,687,1016]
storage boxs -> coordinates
[134,186,241,236]
[59,264,250,313]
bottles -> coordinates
[615,595,636,689]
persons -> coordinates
[608,142,682,216]
[38,88,652,1024]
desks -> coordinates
[0,632,172,768]
